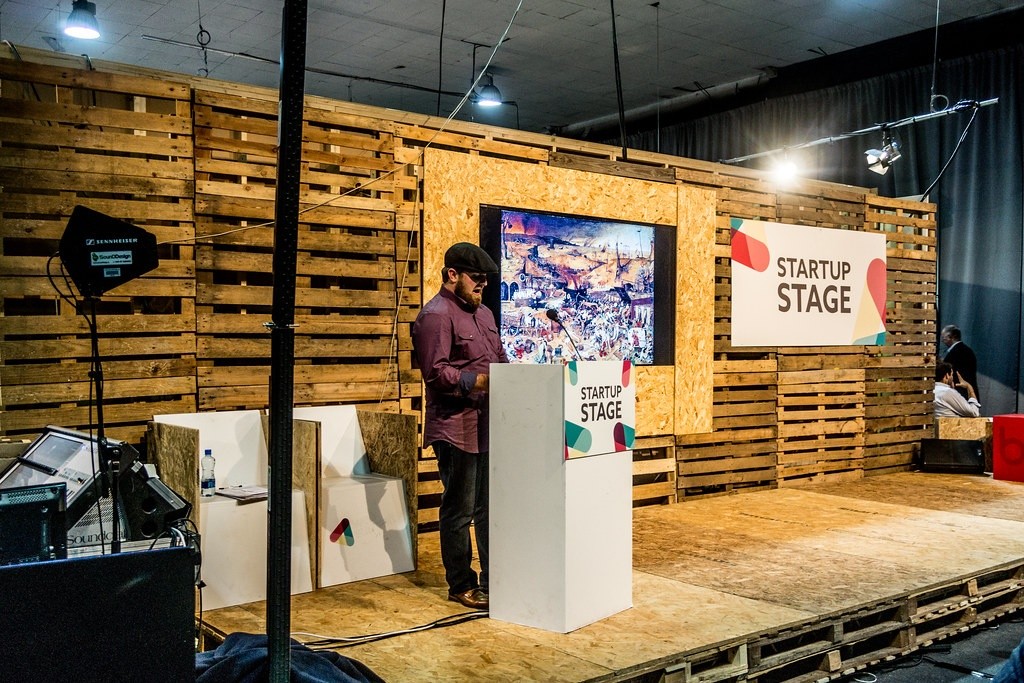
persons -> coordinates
[934,363,980,418]
[412,244,508,609]
[935,325,980,411]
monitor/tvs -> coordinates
[0,425,192,567]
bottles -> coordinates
[201,449,215,497]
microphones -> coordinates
[546,309,583,361]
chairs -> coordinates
[939,417,993,472]
[147,409,317,612]
[263,404,418,590]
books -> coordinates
[216,486,268,502]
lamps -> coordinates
[478,72,502,106]
[864,129,901,175]
[63,0,100,40]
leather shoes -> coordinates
[480,573,489,592]
[449,588,489,608]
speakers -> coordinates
[920,438,985,474]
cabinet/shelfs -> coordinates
[0,546,195,683]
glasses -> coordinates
[465,271,487,284]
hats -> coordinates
[444,242,499,273]
